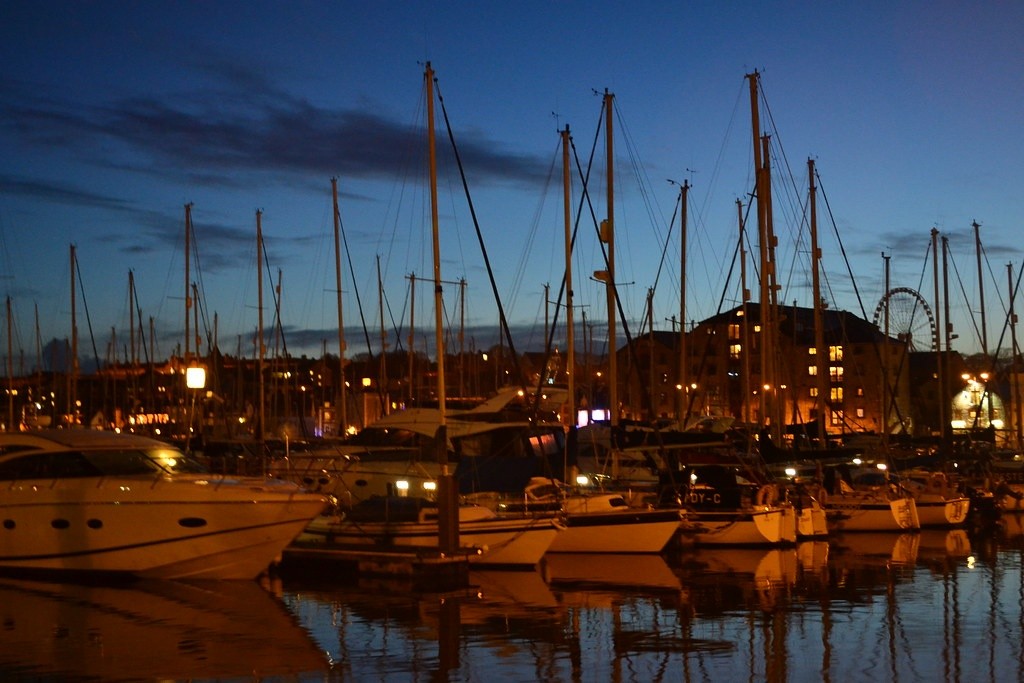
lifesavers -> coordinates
[755,484,774,508]
[817,487,829,506]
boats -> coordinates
[1,427,332,585]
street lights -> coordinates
[960,368,993,436]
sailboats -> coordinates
[303,59,1024,562]
[0,175,397,470]
[338,524,1024,683]
[0,575,345,683]
